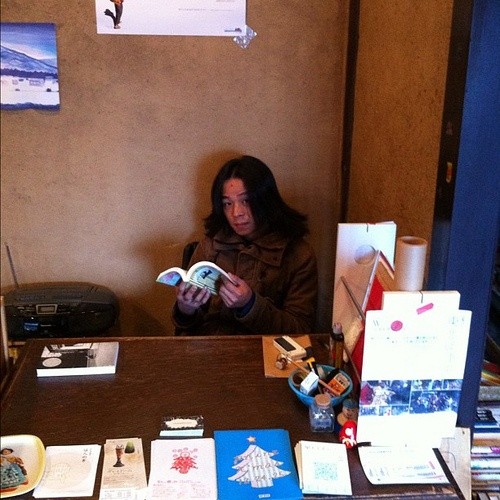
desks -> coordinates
[0,330,461,500]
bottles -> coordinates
[329,322,344,370]
[337,398,359,435]
[309,394,335,432]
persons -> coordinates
[170,154,319,335]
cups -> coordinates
[291,370,307,387]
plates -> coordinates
[0,435,47,499]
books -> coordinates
[155,260,238,296]
[471,359,500,480]
[36,341,119,378]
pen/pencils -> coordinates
[285,357,344,395]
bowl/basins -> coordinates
[288,364,353,407]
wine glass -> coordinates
[113,444,125,467]
